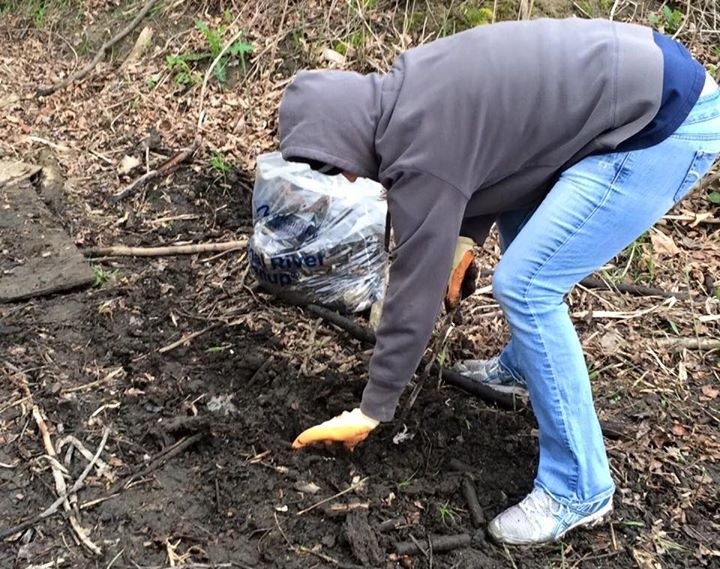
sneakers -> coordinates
[488,488,613,544]
[455,357,530,397]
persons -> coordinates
[279,17,720,551]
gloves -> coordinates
[443,235,478,324]
[292,407,381,452]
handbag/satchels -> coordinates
[250,152,388,313]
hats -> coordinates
[310,159,343,176]
